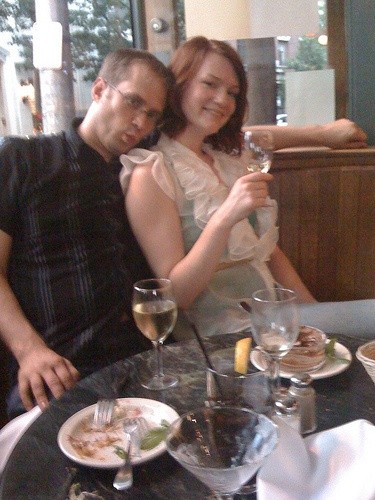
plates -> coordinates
[57,398,182,468]
[251,339,353,380]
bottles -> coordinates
[289,373,317,435]
[273,396,301,435]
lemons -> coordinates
[235,338,252,375]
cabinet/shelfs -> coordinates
[237,36,289,126]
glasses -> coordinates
[110,86,163,124]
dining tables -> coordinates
[0,331,375,500]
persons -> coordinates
[0,46,368,422]
[119,36,375,341]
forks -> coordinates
[94,397,113,424]
[113,418,139,490]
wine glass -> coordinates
[251,288,299,413]
[245,131,274,207]
[165,406,280,500]
[132,278,179,391]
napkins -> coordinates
[256,416,375,500]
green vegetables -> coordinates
[112,419,171,459]
[325,337,351,364]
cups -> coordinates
[206,348,273,415]
